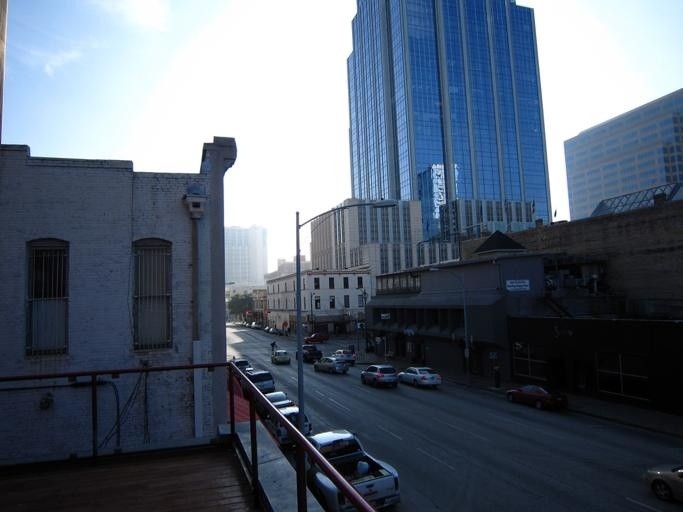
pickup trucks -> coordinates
[303,333,328,344]
[293,429,399,511]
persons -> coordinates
[270,341,275,351]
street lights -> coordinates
[295,197,397,511]
[310,291,315,335]
[429,266,472,382]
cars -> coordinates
[331,350,355,365]
[360,364,397,388]
[641,463,682,502]
[270,350,289,364]
[313,357,348,374]
[397,366,441,388]
[504,383,568,410]
[239,369,274,399]
[240,321,278,334]
[229,358,249,379]
[295,345,322,362]
[257,391,311,446]
